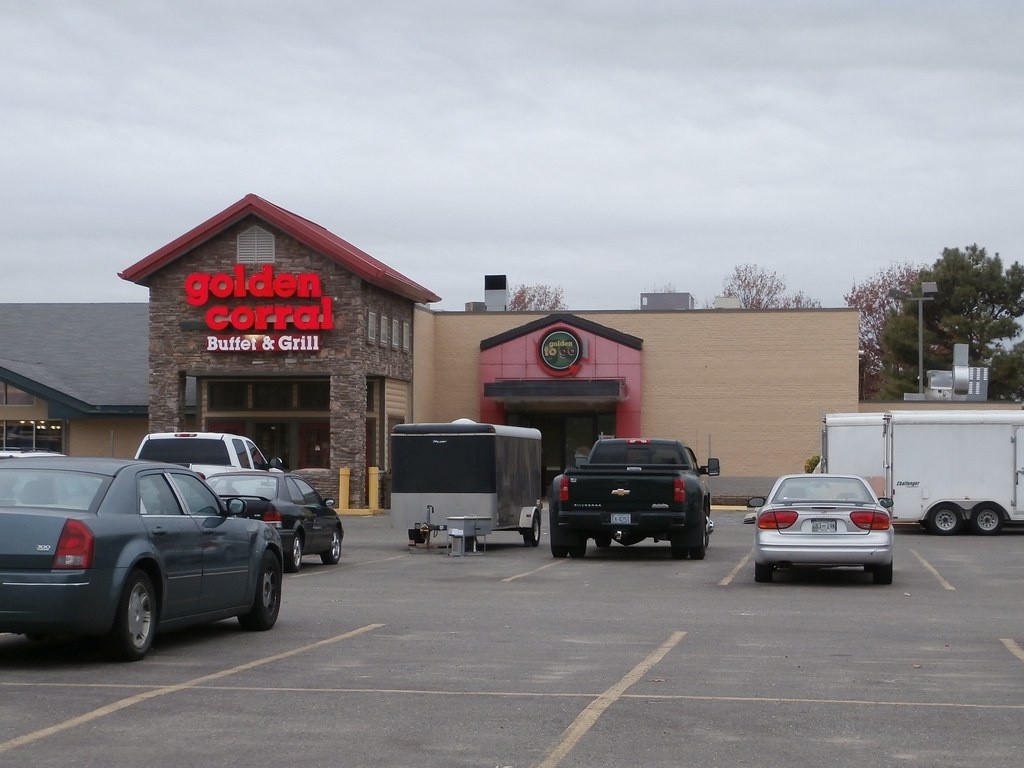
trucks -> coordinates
[818,408,1024,537]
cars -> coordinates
[744,472,895,585]
[201,470,345,573]
[0,455,287,663]
[0,450,67,460]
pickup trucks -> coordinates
[546,434,721,560]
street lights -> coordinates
[916,281,938,392]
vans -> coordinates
[127,430,285,520]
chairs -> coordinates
[598,450,628,464]
[661,455,678,464]
[18,482,67,504]
[783,487,808,498]
